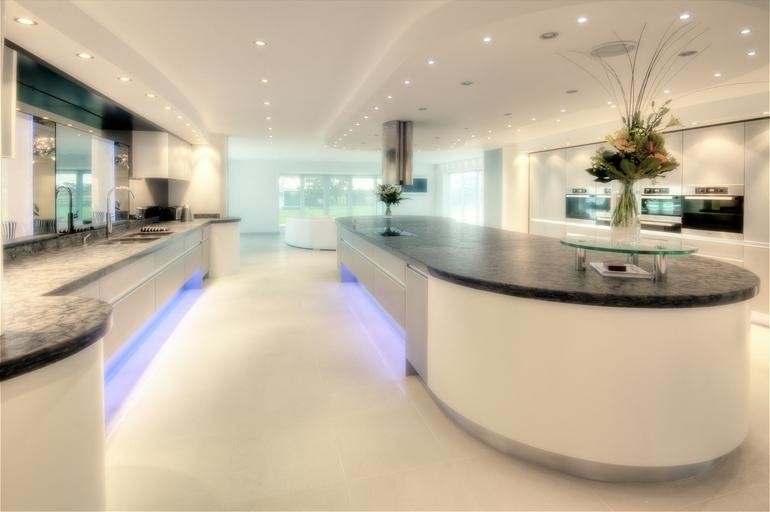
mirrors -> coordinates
[32,116,131,237]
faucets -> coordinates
[106,186,136,233]
[55,185,73,233]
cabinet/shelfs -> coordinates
[132,130,195,184]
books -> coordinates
[590,261,652,279]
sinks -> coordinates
[96,238,159,244]
[124,232,174,238]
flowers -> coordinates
[550,17,768,228]
[372,182,415,208]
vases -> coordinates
[384,203,391,218]
